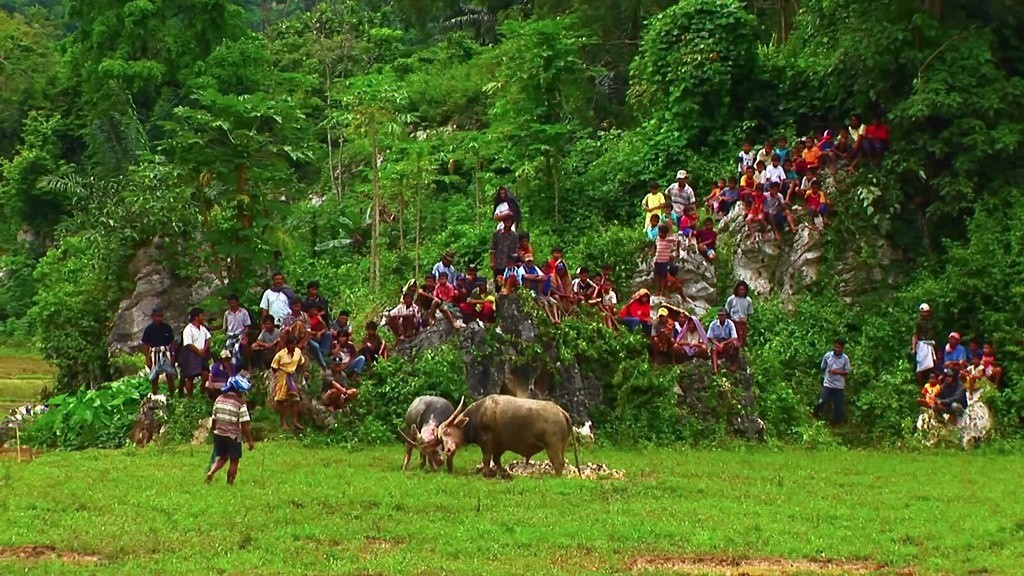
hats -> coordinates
[948,333,960,343]
[443,250,455,262]
[657,307,668,316]
[456,273,467,281]
[555,261,566,271]
[717,308,729,314]
[330,356,343,364]
[523,253,534,262]
[465,264,479,275]
[676,170,687,179]
[919,303,931,311]
[151,307,166,316]
[220,349,232,359]
[475,277,488,286]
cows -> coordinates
[397,393,583,482]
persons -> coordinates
[207,375,254,486]
[489,186,621,333]
[702,113,890,244]
[912,302,1001,426]
[812,339,850,426]
[641,170,717,297]
[140,273,389,433]
[617,280,754,374]
[380,252,495,349]
